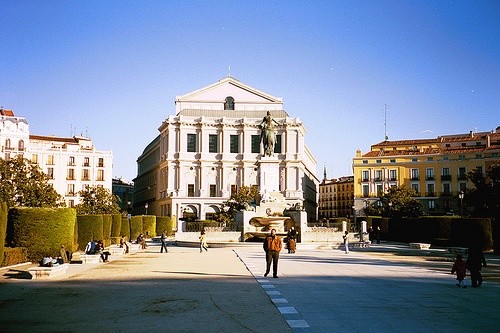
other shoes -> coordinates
[462,286,467,288]
[273,275,278,278]
[264,273,267,277]
[456,284,460,287]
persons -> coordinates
[286,226,297,254]
[263,230,282,279]
[120,236,130,254]
[136,234,148,250]
[450,242,488,288]
[85,239,109,262]
[342,231,350,253]
[257,110,281,144]
[199,231,209,254]
[369,225,383,244]
[161,229,170,253]
